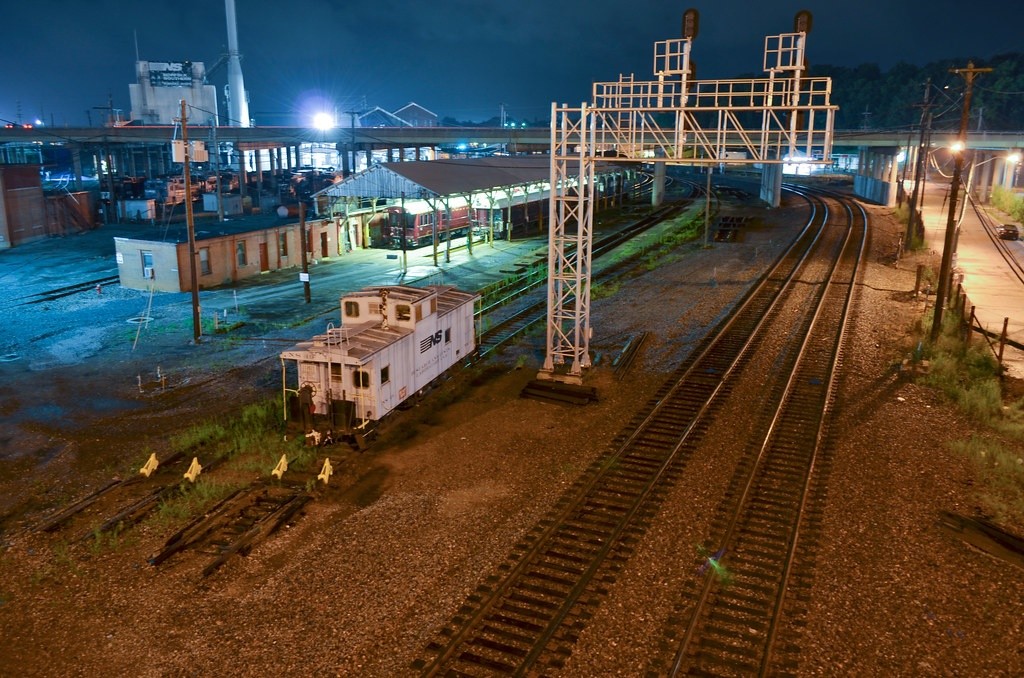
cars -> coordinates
[996,223,1019,240]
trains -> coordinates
[381,169,628,250]
